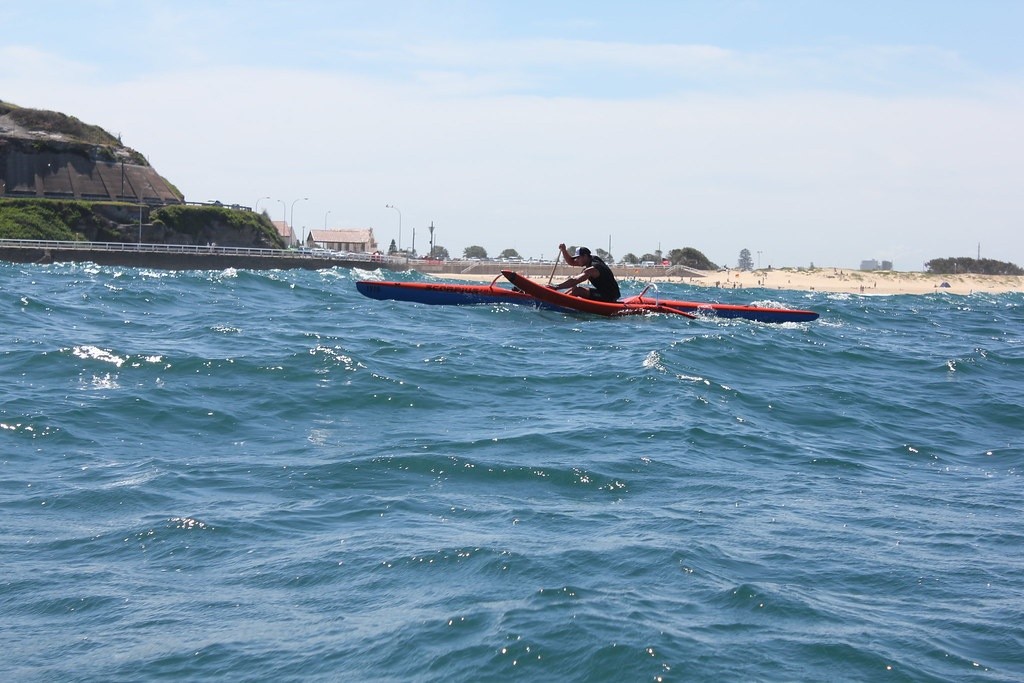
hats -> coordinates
[570,247,591,258]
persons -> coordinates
[642,261,1024,302]
[545,243,621,303]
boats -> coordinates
[616,295,819,322]
[356,279,578,314]
[500,270,698,320]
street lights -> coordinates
[139,185,152,248]
[323,211,331,249]
[290,197,308,249]
[385,204,402,257]
[255,196,270,213]
[277,199,285,241]
